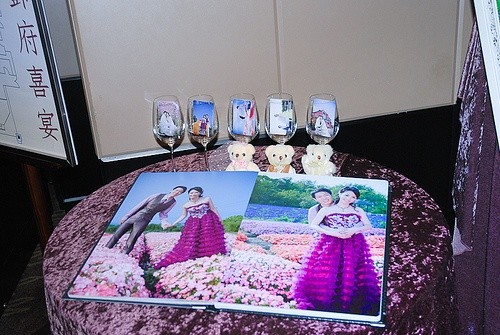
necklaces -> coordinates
[190,199,199,203]
[337,204,351,211]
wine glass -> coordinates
[186,94,220,171]
[264,93,298,145]
[152,96,186,173]
[305,93,339,145]
[227,93,260,143]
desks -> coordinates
[42,145,452,335]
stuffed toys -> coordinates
[301,145,336,176]
[226,144,260,171]
[266,145,296,175]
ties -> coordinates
[160,199,167,204]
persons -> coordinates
[153,187,227,269]
[105,186,187,255]
[158,104,331,137]
[308,188,364,235]
[290,186,381,316]
